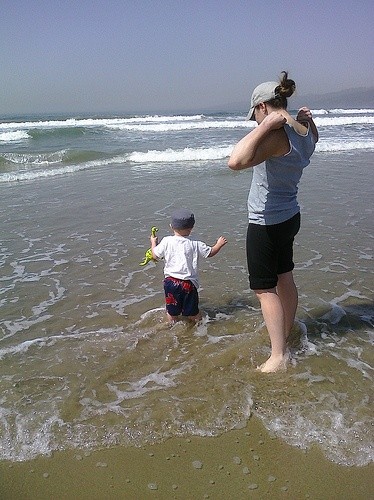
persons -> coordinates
[149,208,227,322]
[229,71,321,375]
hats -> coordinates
[171,209,195,230]
[244,81,282,121]
